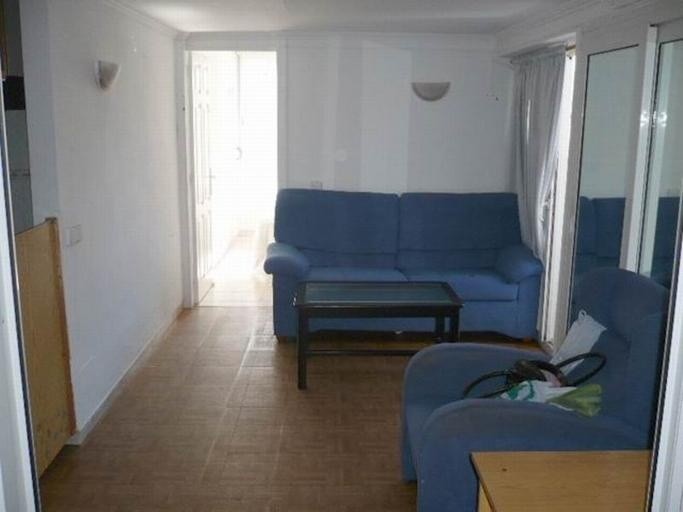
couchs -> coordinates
[263,188,542,344]
[569,196,679,333]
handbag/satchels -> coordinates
[461,351,606,403]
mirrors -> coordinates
[571,40,683,289]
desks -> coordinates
[468,450,652,512]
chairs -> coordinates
[399,266,672,510]
